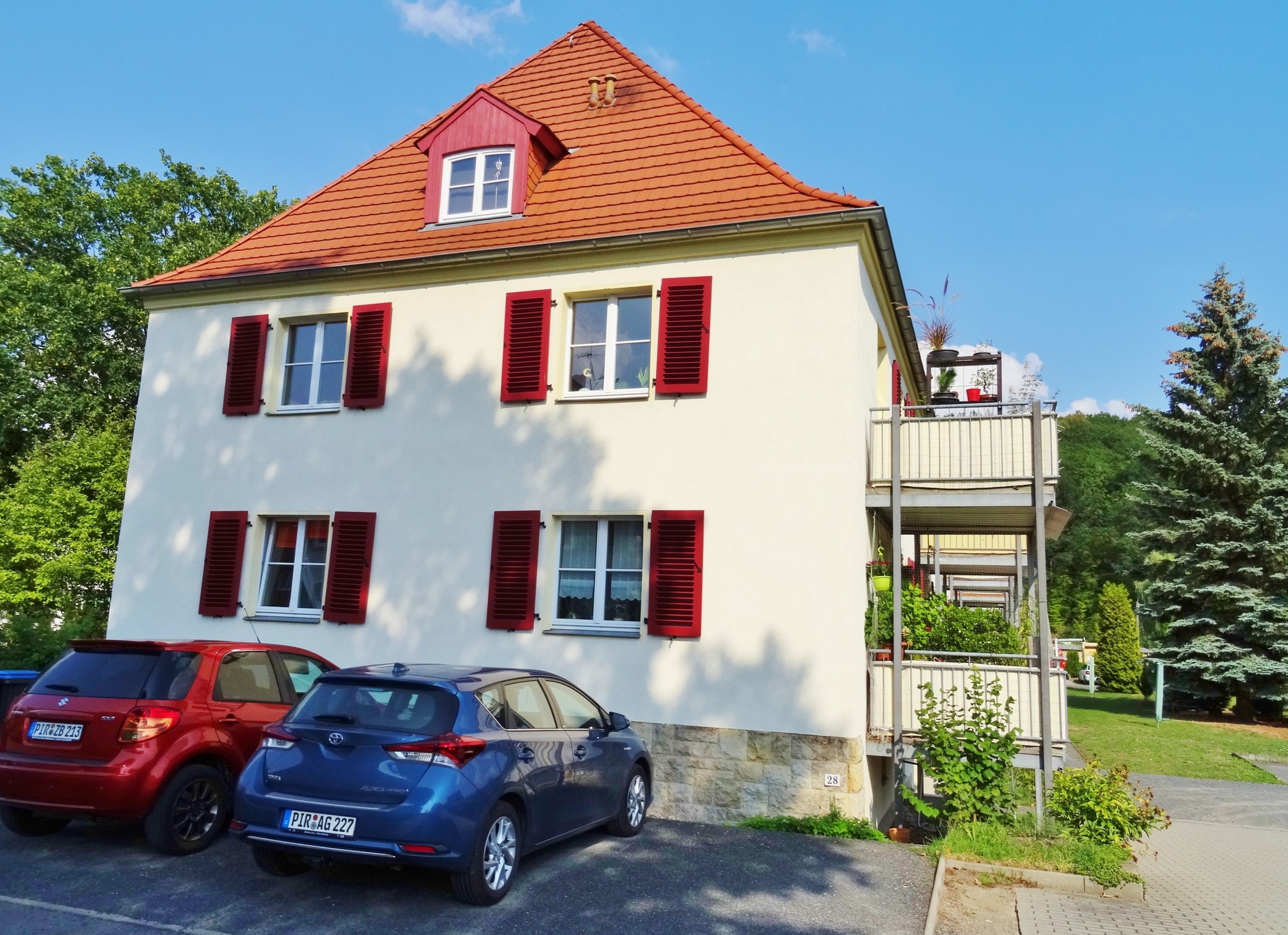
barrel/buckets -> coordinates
[966,388,981,401]
[889,828,909,844]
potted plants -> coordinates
[865,582,949,661]
[973,338,993,359]
[933,368,959,403]
[890,274,960,357]
[968,367,999,402]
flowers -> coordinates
[867,561,894,576]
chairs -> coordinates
[229,664,264,697]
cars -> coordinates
[0,670,41,726]
[230,662,655,906]
[1078,663,1100,685]
[0,637,388,855]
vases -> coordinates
[966,388,982,401]
[870,577,892,591]
